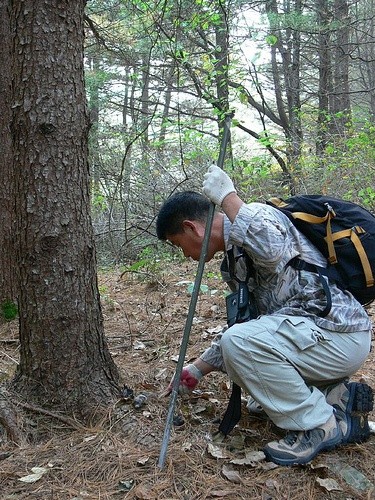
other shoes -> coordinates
[326,382,373,442]
[264,407,344,466]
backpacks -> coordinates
[235,195,375,318]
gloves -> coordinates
[202,165,237,207]
[167,364,203,395]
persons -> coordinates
[157,164,373,466]
